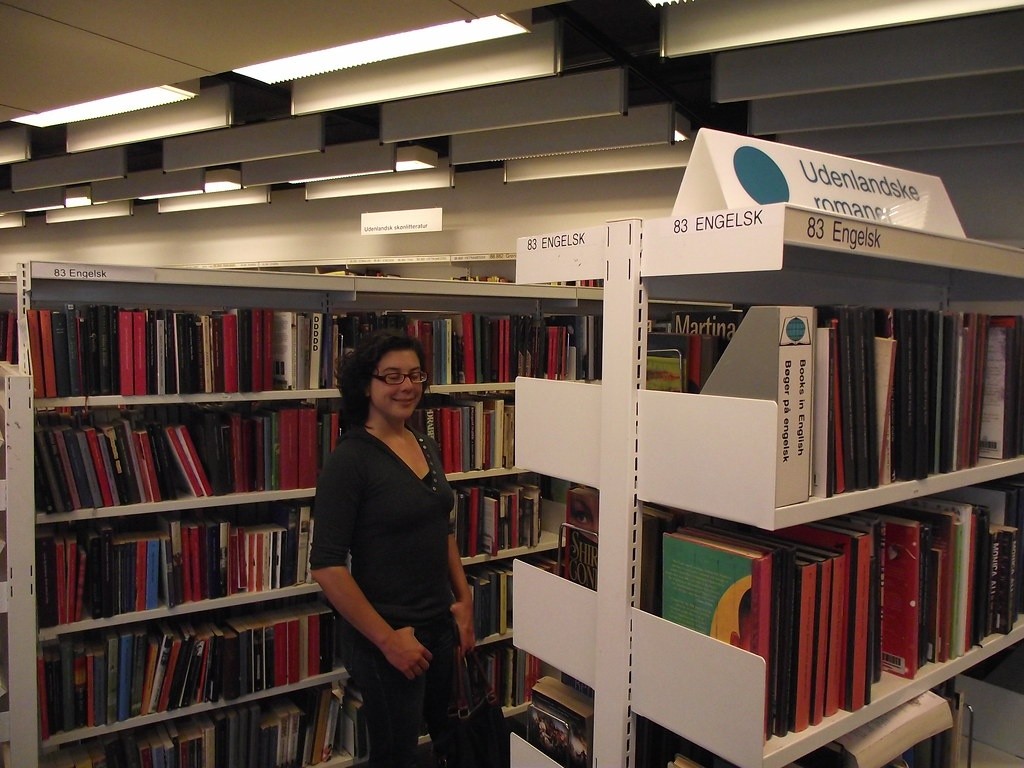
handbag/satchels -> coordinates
[438,651,510,768]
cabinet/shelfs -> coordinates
[0,210,1024,768]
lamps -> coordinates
[205,168,241,194]
[66,186,93,207]
[229,10,532,88]
[396,144,438,173]
[9,76,200,129]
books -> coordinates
[647,306,1024,768]
[0,276,603,768]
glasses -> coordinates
[372,372,427,385]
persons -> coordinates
[309,328,475,768]
[571,494,599,541]
